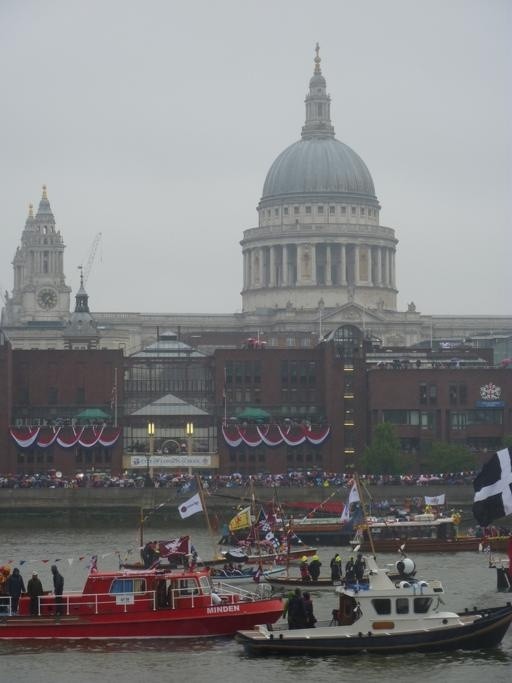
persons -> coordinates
[141,541,158,568]
[0,563,65,616]
[88,554,98,574]
[282,588,319,629]
[300,552,366,583]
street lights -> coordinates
[148,421,154,479]
[186,421,193,477]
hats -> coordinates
[302,556,320,562]
[334,556,343,562]
[31,571,39,576]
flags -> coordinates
[473,447,512,528]
[179,492,203,518]
[229,506,282,546]
[159,534,191,558]
[339,481,364,527]
